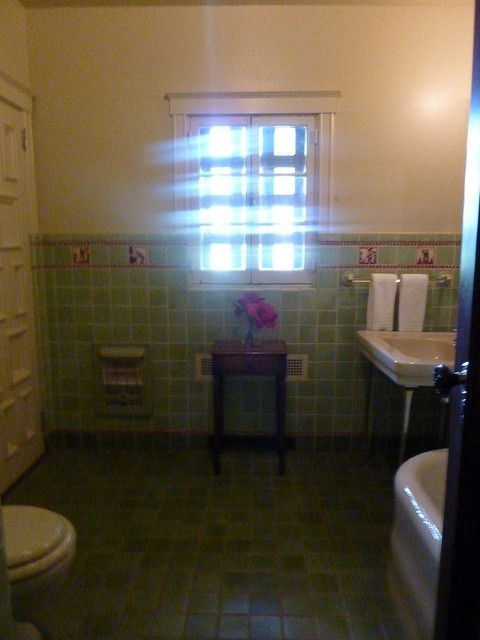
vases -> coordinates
[243,322,261,347]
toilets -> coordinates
[0,504,77,640]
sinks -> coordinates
[382,337,454,363]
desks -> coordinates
[211,338,292,477]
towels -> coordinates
[368,274,397,333]
[399,273,429,332]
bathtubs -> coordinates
[388,448,448,634]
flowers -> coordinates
[233,292,277,346]
